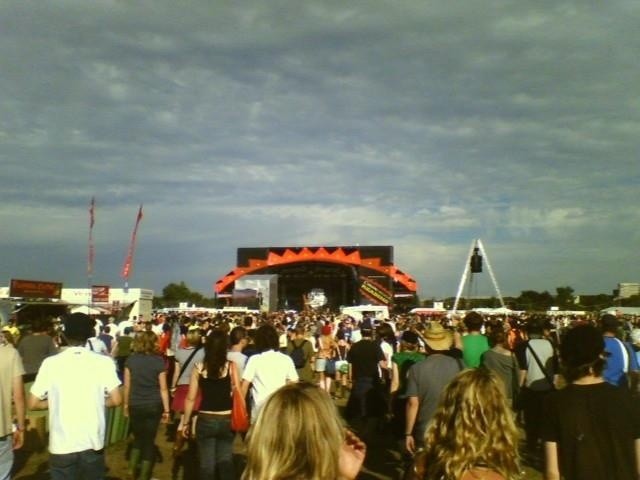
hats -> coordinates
[415,320,454,351]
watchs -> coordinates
[17,426,26,433]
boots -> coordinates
[127,448,141,479]
[140,459,151,480]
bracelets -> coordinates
[164,412,171,414]
[183,422,191,426]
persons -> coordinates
[177,308,357,341]
[480,331,520,413]
[388,331,428,453]
[358,310,467,353]
[348,322,389,431]
[239,325,298,426]
[286,326,315,382]
[598,314,640,387]
[171,328,205,454]
[408,368,543,480]
[332,332,351,399]
[111,328,132,385]
[181,331,241,480]
[405,322,461,480]
[131,309,180,359]
[542,325,640,480]
[0,314,25,480]
[516,322,558,453]
[17,317,57,449]
[2,319,21,347]
[118,316,132,337]
[123,331,170,480]
[86,317,118,356]
[225,326,251,381]
[483,310,640,351]
[29,312,123,480]
[236,381,367,480]
[460,311,493,368]
[256,292,265,306]
[315,325,341,396]
[53,315,67,346]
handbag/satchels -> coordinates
[176,347,201,386]
[325,357,336,376]
[228,363,249,432]
[525,343,548,378]
